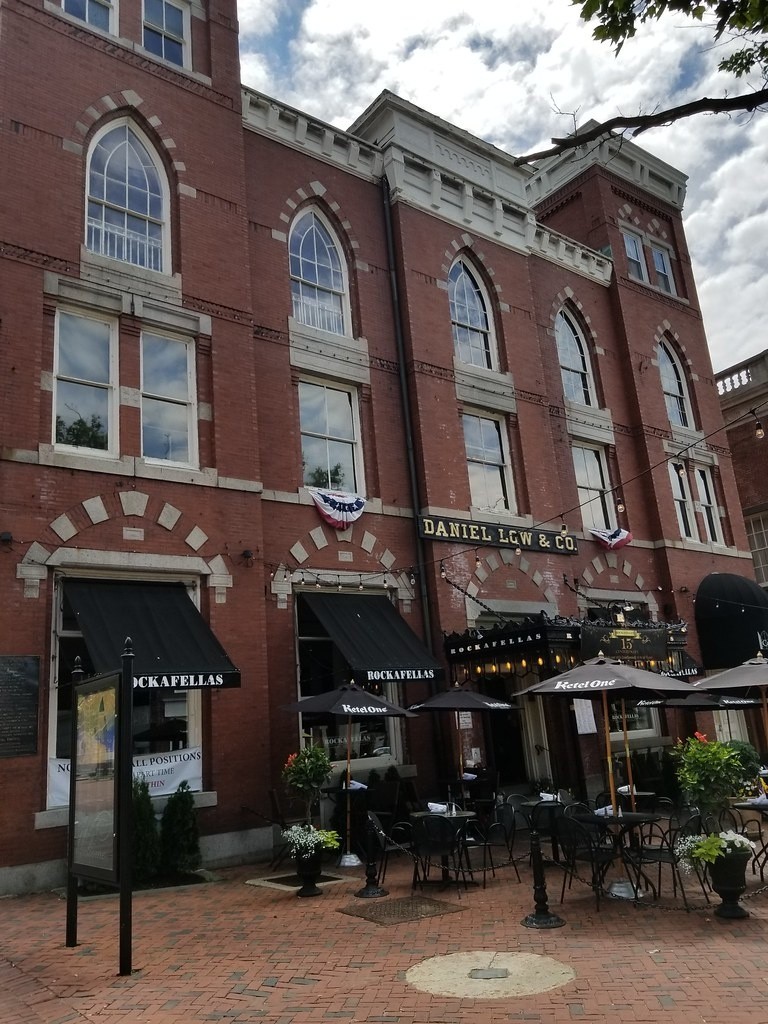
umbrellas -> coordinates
[622,693,722,838]
[688,693,765,741]
[510,650,709,881]
[401,681,528,835]
[279,678,420,855]
[688,651,768,756]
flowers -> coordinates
[727,778,768,797]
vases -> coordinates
[725,795,768,837]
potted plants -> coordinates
[676,830,756,919]
[280,825,341,898]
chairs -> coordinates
[263,743,768,915]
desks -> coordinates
[732,802,768,882]
[409,810,477,890]
[321,786,377,866]
[520,800,582,864]
[600,791,657,863]
[569,811,661,901]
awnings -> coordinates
[60,577,241,692]
[694,572,768,670]
[298,592,445,685]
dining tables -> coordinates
[438,778,480,810]
